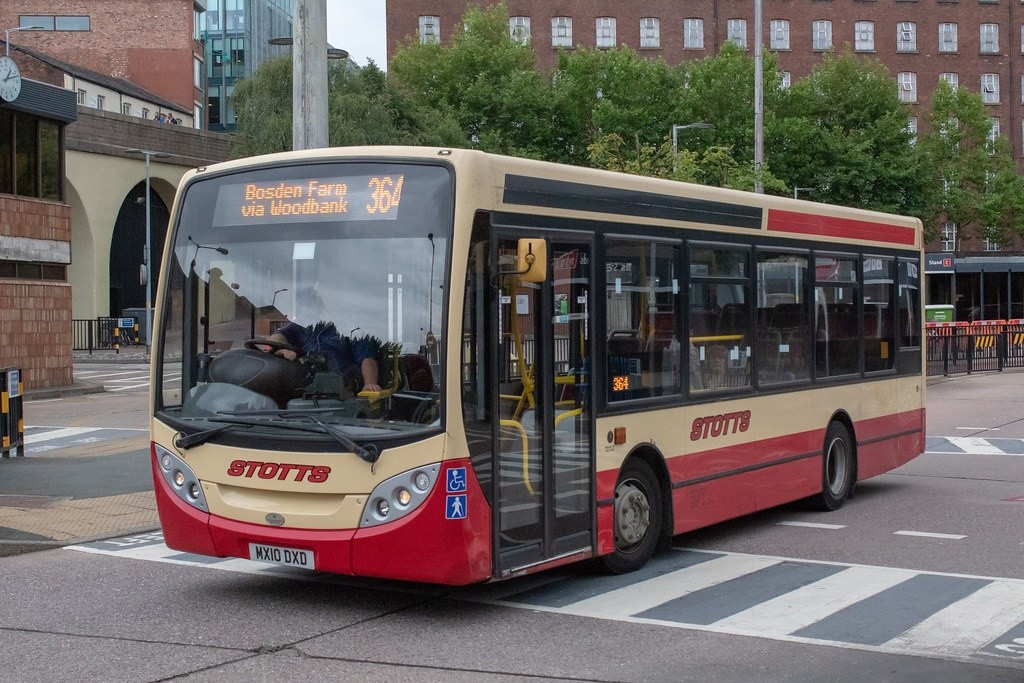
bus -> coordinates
[123,144,929,588]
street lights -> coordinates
[794,186,815,199]
[670,121,715,176]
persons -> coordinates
[637,302,890,395]
[268,275,382,391]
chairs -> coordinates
[560,303,911,399]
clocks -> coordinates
[0,55,22,102]
